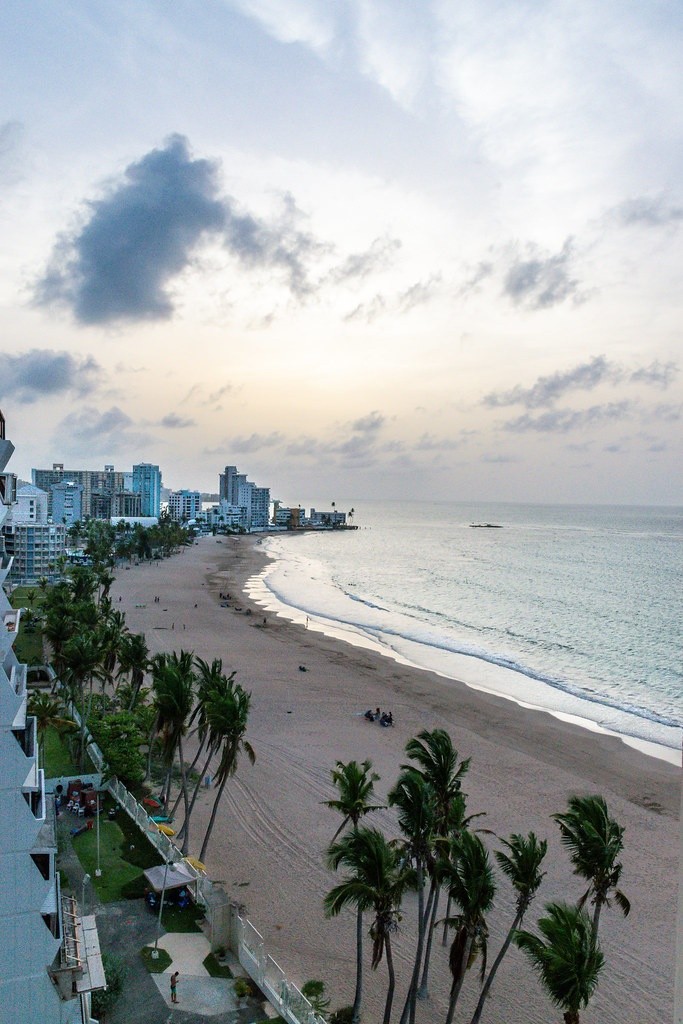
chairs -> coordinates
[66,800,84,819]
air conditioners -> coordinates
[0,472,18,505]
[0,536,5,552]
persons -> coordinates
[116,539,267,629]
[364,707,393,727]
[170,971,179,1003]
